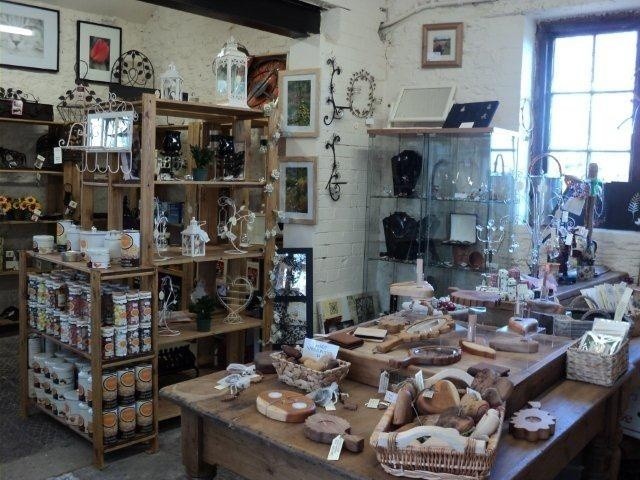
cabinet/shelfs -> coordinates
[361,126,518,316]
[73,92,280,432]
[17,247,158,470]
[0,116,83,332]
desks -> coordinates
[156,262,640,480]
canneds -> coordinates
[27,268,155,361]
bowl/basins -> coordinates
[87,248,111,269]
[467,250,483,268]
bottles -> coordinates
[56,218,74,252]
[28,270,161,449]
[80,227,108,260]
[68,225,81,251]
[104,229,123,264]
[32,235,54,255]
[121,228,140,267]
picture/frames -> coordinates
[275,155,318,224]
[277,67,321,137]
[0,0,60,73]
[77,20,122,84]
[421,22,462,66]
[388,84,456,123]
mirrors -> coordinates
[256,248,314,350]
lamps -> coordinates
[213,34,251,109]
[159,61,183,102]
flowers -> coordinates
[0,194,45,222]
